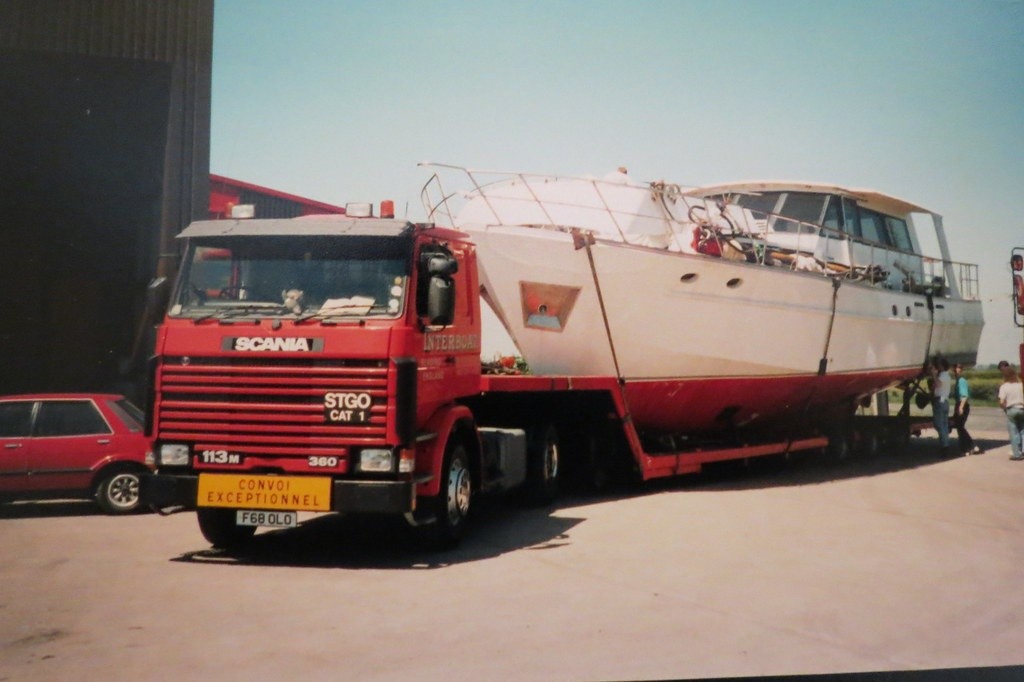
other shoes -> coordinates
[960,445,979,456]
[1009,452,1024,460]
[937,446,951,457]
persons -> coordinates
[954,364,980,456]
[998,361,1024,461]
[930,361,952,452]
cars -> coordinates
[0,391,186,515]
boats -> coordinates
[417,132,984,376]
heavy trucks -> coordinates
[140,175,951,554]
